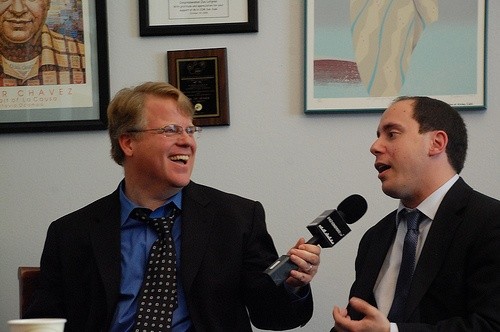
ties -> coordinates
[128,206,181,332]
[388,208,428,324]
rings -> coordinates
[307,266,313,272]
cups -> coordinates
[7,318,67,332]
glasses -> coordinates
[125,124,203,137]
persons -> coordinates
[19,82,313,332]
[329,96,500,332]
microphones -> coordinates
[263,194,369,289]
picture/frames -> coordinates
[168,47,230,127]
[304,0,487,113]
[0,0,110,133]
[139,0,258,37]
